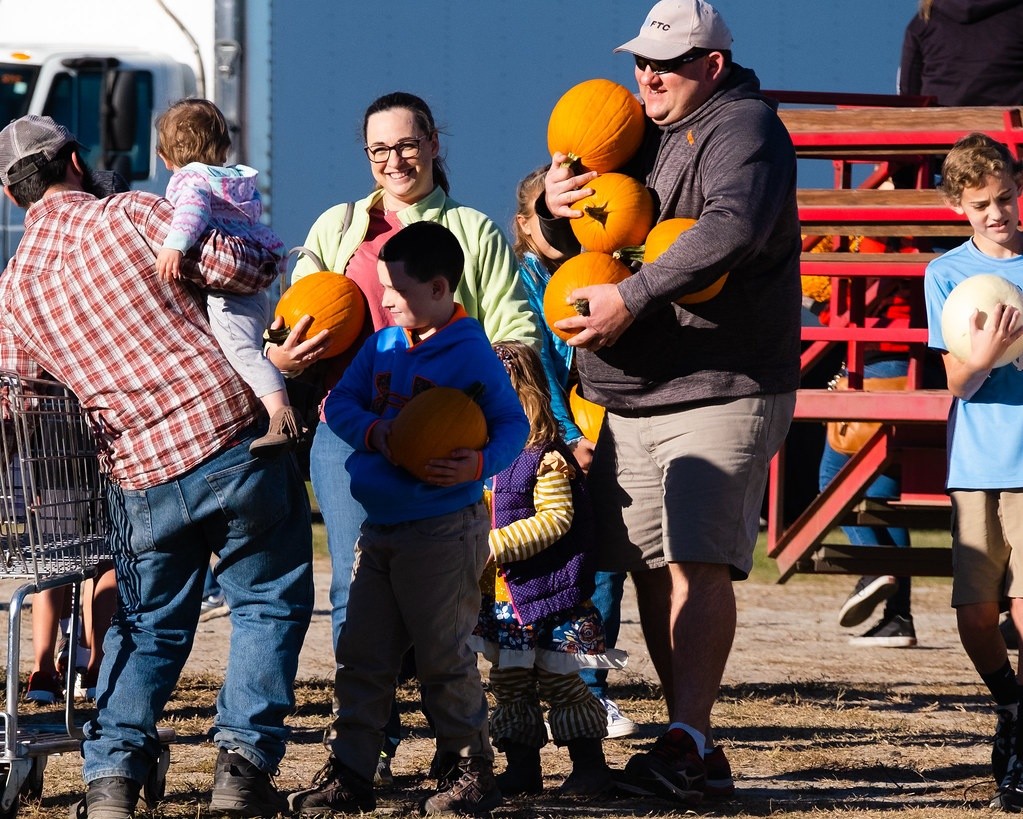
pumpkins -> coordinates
[941,273,1023,369]
[261,271,364,359]
[566,173,652,254]
[566,383,605,445]
[800,233,865,303]
[543,251,636,348]
[547,79,645,176]
[612,218,730,304]
[386,380,488,484]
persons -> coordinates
[533,0,803,799]
[924,131,1022,814]
[263,92,542,781]
[512,162,639,740]
[899,0,1023,108]
[799,236,930,647]
[288,221,530,812]
[465,340,614,792]
[1,114,314,819]
[25,568,118,704]
[198,548,231,622]
[156,100,310,456]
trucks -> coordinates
[0,38,199,279]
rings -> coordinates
[306,353,313,363]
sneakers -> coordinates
[199,590,231,622]
[989,749,1023,813]
[85,674,98,700]
[73,666,88,697]
[991,700,1023,788]
[286,762,377,814]
[849,611,916,647]
[598,697,640,739]
[420,756,503,816]
[57,636,70,673]
[208,746,289,818]
[839,575,899,627]
[24,671,65,704]
[69,776,142,819]
[626,728,708,803]
[610,745,735,798]
[372,751,393,789]
[429,751,466,780]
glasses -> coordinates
[363,133,431,163]
[633,49,712,75]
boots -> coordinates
[553,737,614,800]
[495,743,543,794]
[249,406,313,458]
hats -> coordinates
[613,0,734,61]
[0,114,91,187]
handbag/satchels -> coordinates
[826,372,908,455]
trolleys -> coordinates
[0,368,178,819]
